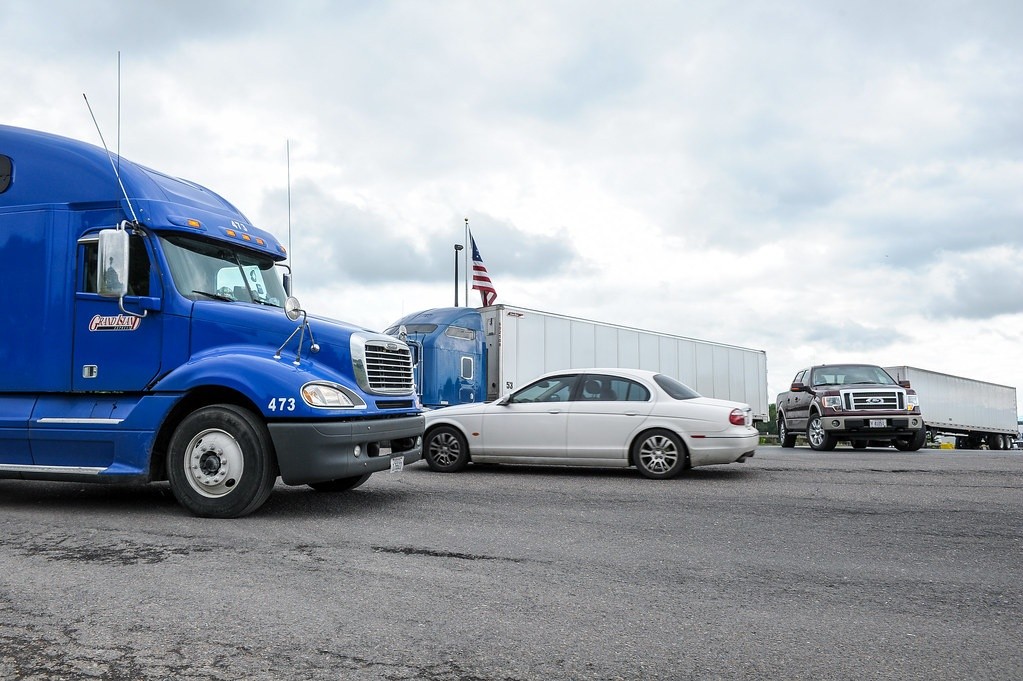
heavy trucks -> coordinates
[1,126,425,518]
[381,304,770,424]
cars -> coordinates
[421,366,759,477]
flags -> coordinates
[468,230,497,307]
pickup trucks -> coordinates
[773,363,928,451]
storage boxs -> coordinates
[939,435,956,448]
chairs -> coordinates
[586,380,600,400]
[815,375,827,384]
